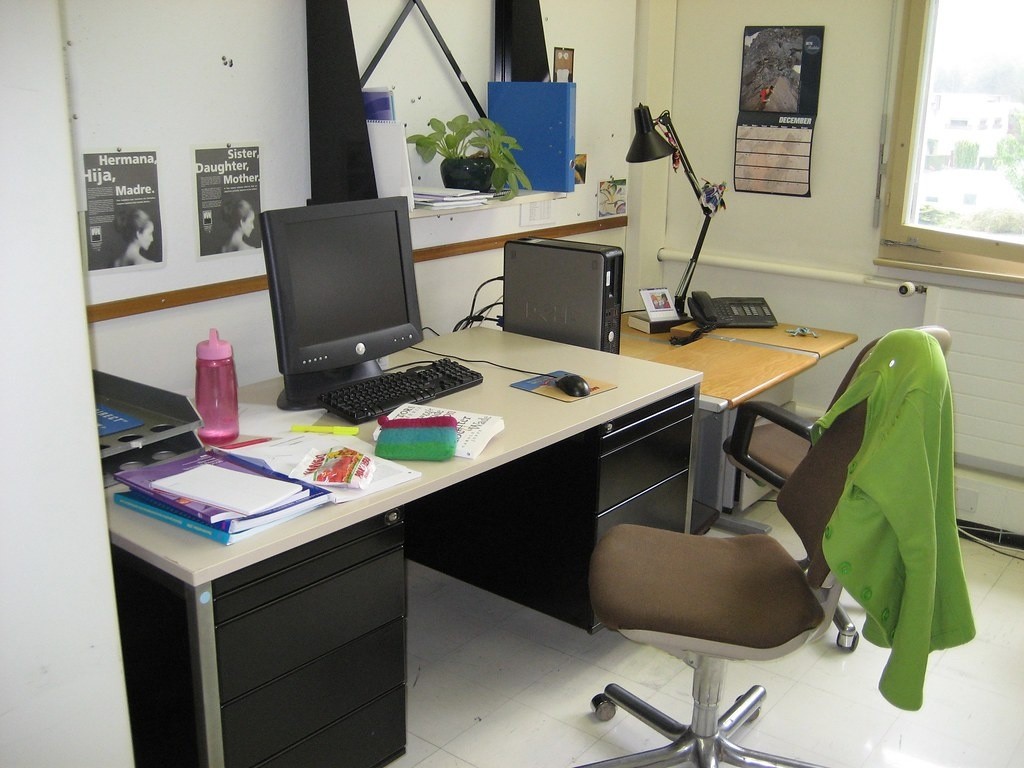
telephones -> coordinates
[687,290,778,329]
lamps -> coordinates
[626,104,728,332]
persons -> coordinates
[217,199,257,254]
[661,293,670,308]
[651,294,662,309]
[760,86,774,102]
[110,208,155,266]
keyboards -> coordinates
[318,357,483,425]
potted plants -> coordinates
[405,114,532,202]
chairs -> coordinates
[570,330,976,768]
[722,326,950,651]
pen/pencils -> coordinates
[219,438,273,449]
[291,425,359,436]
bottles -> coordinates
[192,330,240,446]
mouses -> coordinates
[555,373,590,397]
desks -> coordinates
[608,306,859,534]
[103,324,705,768]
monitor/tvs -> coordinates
[256,195,426,411]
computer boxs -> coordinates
[502,236,625,354]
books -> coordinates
[627,310,694,334]
[114,451,332,546]
[413,187,510,210]
[373,403,505,461]
[367,120,416,210]
